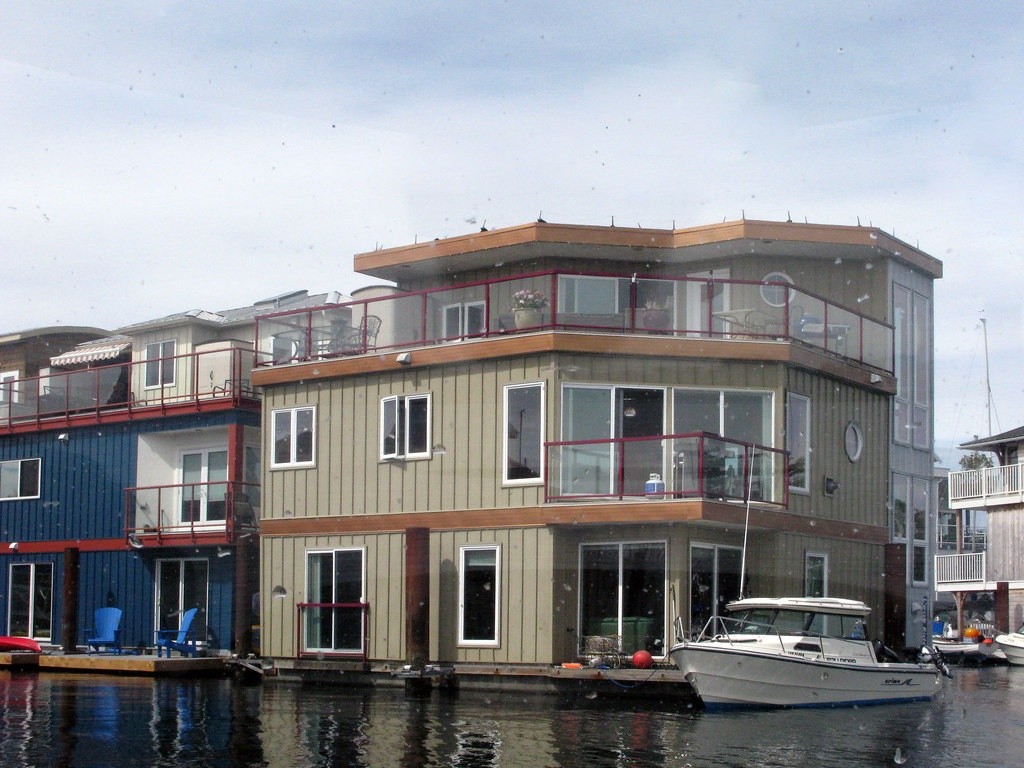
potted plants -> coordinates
[641,282,673,334]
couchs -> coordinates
[585,616,661,657]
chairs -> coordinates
[130,392,147,407]
[213,380,249,396]
[43,386,74,418]
[81,607,124,656]
[765,305,804,343]
[154,608,199,657]
[339,315,384,355]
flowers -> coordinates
[507,289,551,312]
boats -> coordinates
[995,623,1024,666]
[666,443,956,708]
[931,621,999,666]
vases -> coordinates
[512,308,542,333]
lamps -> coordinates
[871,373,883,384]
[396,353,411,364]
[623,407,636,417]
[8,542,19,550]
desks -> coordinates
[713,307,778,340]
[801,323,851,356]
[76,381,127,413]
[271,325,364,364]
